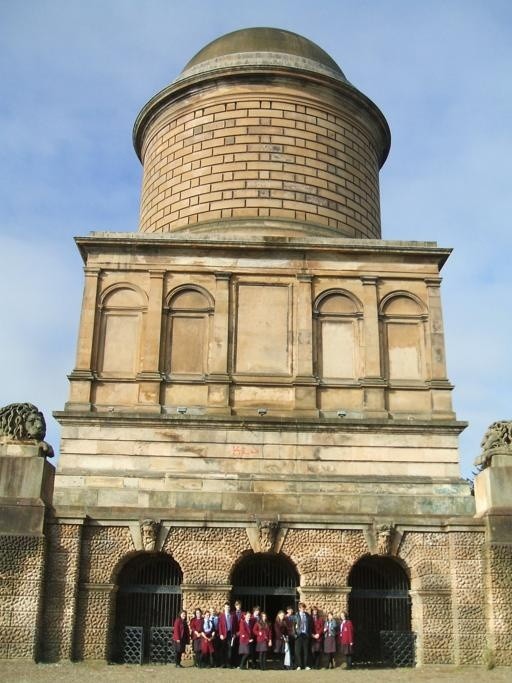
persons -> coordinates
[172,601,356,670]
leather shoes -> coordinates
[193,662,269,669]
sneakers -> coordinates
[297,666,301,669]
[305,666,312,669]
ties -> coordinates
[227,614,230,630]
[302,614,304,630]
